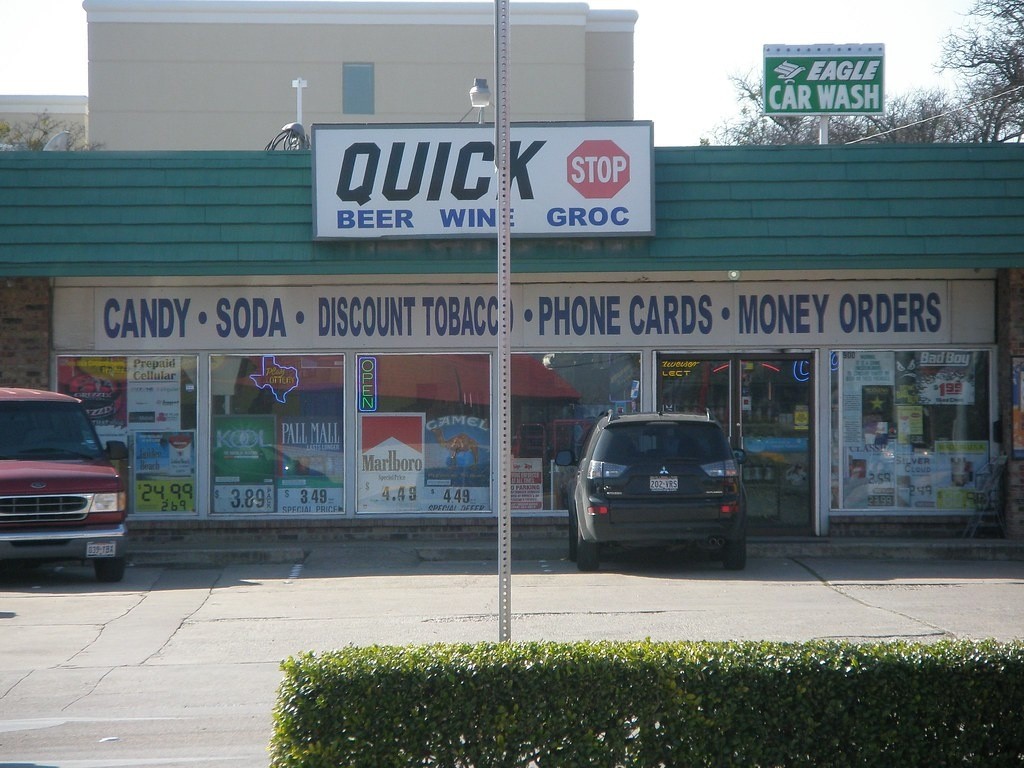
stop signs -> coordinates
[565,138,632,201]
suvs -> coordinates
[554,407,748,572]
[0,386,131,583]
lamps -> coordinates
[470,77,495,124]
